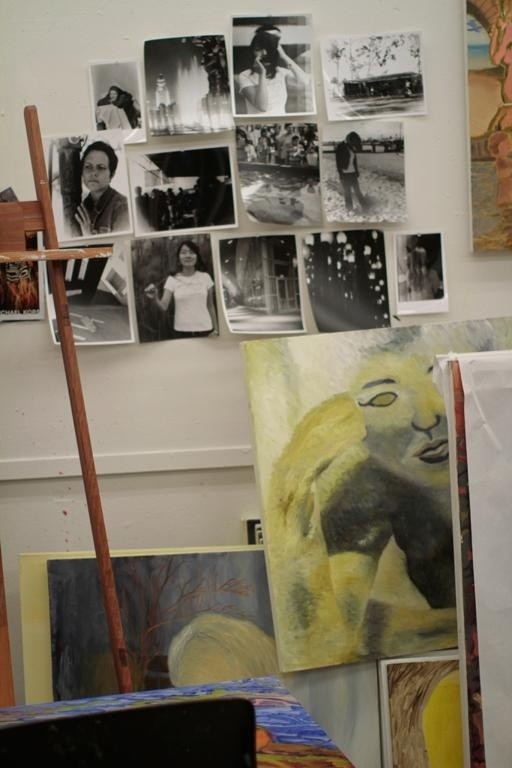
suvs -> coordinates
[145,650,171,689]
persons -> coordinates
[234,22,315,120]
[93,86,143,134]
[71,139,129,237]
[408,245,442,305]
[143,241,218,339]
[135,172,235,229]
[332,132,372,214]
[234,129,318,169]
[268,321,493,663]
